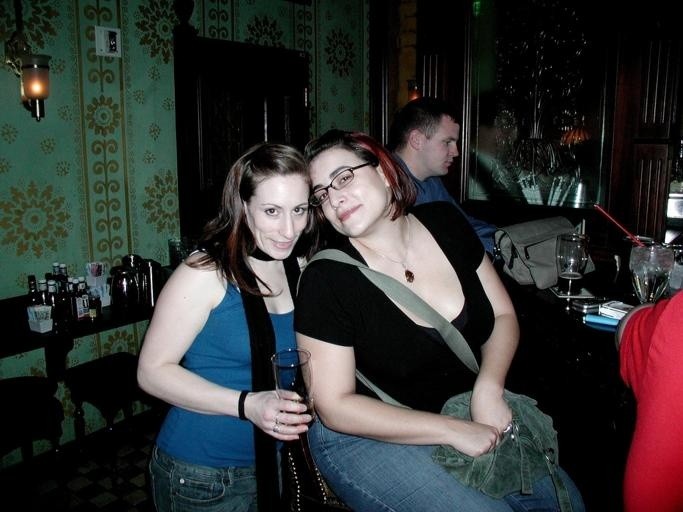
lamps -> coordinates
[4,29,50,123]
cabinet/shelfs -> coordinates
[367,0,640,232]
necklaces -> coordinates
[355,214,414,282]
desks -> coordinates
[493,239,683,512]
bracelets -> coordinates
[239,390,251,421]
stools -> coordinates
[0,374,66,512]
[69,348,168,495]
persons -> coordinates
[295,130,586,512]
[386,96,501,261]
[136,143,327,512]
[614,289,683,512]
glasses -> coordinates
[308,162,371,206]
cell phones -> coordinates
[573,296,604,313]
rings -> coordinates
[273,423,283,432]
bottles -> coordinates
[25,261,101,336]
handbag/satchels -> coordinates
[431,388,559,501]
[493,216,596,290]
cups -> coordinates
[628,242,675,307]
[167,237,197,271]
[271,347,317,429]
[554,232,590,295]
